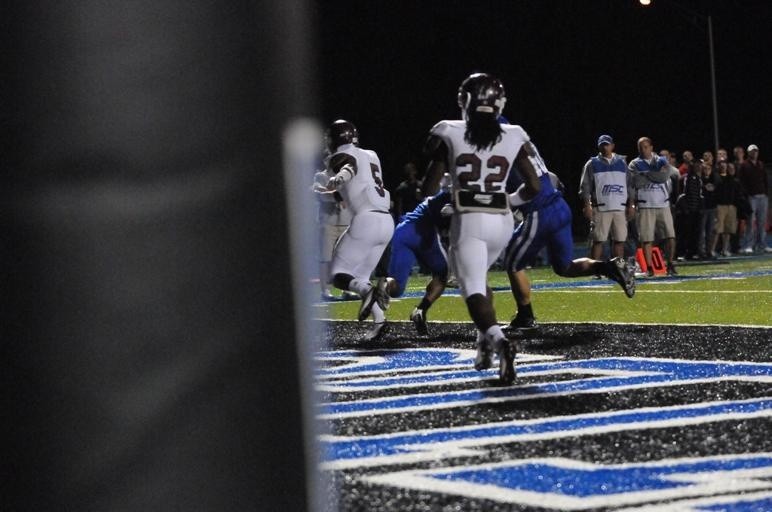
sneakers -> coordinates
[647,266,654,277]
[409,305,428,338]
[666,262,681,277]
[677,246,772,262]
[474,339,493,371]
[500,312,540,330]
[606,256,636,298]
[357,276,390,344]
[319,291,343,301]
[493,336,517,385]
[342,291,362,300]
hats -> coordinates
[747,144,759,153]
[598,135,612,147]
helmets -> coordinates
[457,72,507,122]
[324,119,359,154]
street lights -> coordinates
[639,0,721,158]
